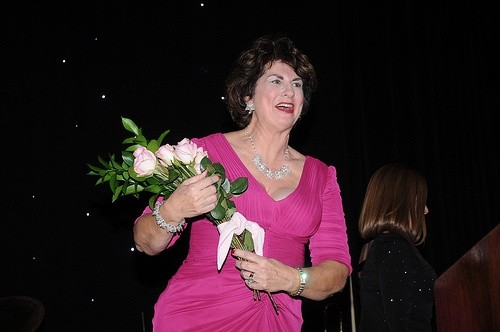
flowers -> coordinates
[86,116,279,315]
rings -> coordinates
[248,274,254,284]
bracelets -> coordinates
[152,201,185,233]
[288,266,306,297]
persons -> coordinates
[132,37,353,332]
[358,163,436,332]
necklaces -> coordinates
[244,129,291,180]
